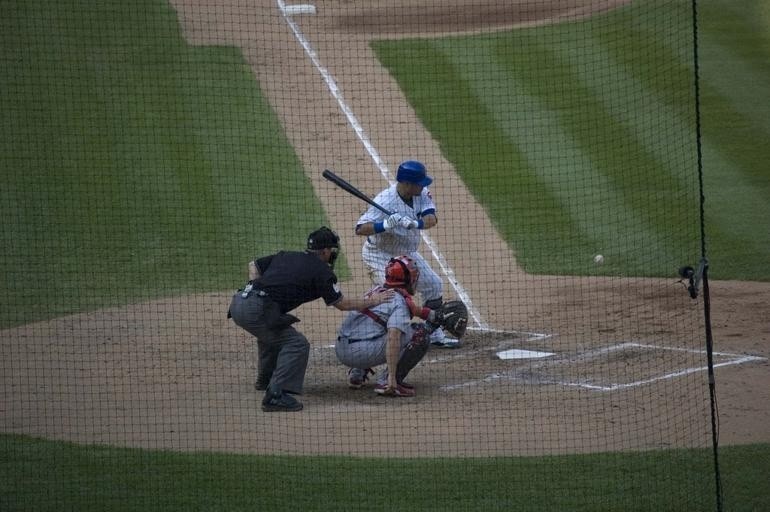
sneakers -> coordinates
[374,374,417,398]
[345,365,368,389]
[260,391,304,414]
[254,372,271,391]
[429,326,460,349]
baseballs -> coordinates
[594,255,604,264]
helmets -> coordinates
[383,254,421,297]
[304,225,341,271]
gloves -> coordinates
[400,215,424,230]
[373,212,402,234]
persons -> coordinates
[230,226,394,412]
[356,159,460,349]
[334,253,444,399]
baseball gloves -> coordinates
[435,301,468,337]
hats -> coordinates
[396,159,433,188]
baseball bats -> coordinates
[322,169,415,230]
